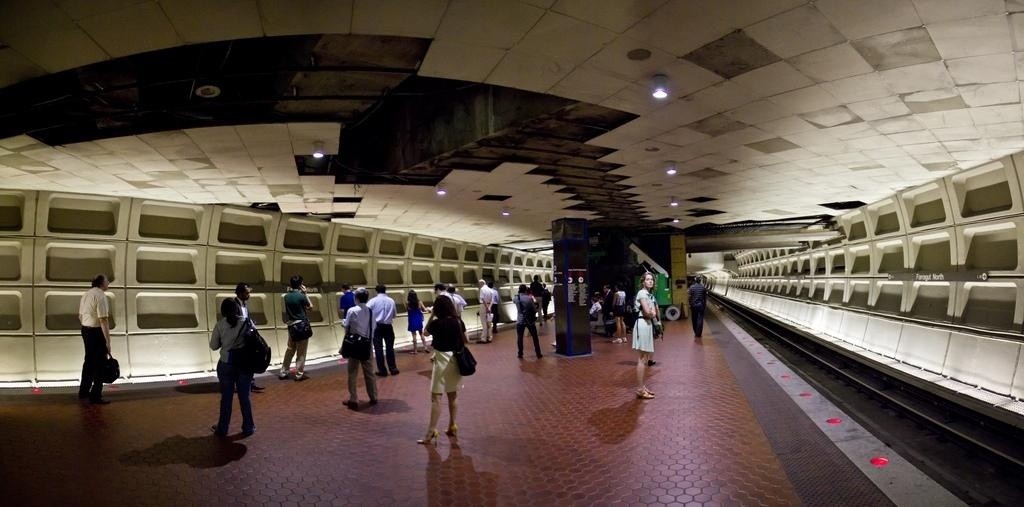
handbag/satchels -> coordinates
[288,320,312,343]
[523,307,538,325]
[454,347,478,377]
[102,359,120,384]
[653,322,664,338]
[623,313,639,330]
[339,332,369,360]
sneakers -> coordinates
[409,352,418,354]
[91,399,111,404]
[611,338,627,344]
[343,400,359,409]
[253,385,265,390]
[476,341,487,344]
[78,392,91,399]
[376,370,400,376]
[424,348,430,353]
[278,373,308,381]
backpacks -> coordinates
[234,315,270,373]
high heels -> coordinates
[417,431,439,444]
[445,424,457,435]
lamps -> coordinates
[502,207,512,216]
[437,181,448,196]
[652,74,680,223]
[313,141,324,159]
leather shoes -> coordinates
[648,360,655,367]
[637,387,655,399]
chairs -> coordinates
[588,311,606,335]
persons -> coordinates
[631,271,655,399]
[689,275,708,338]
[476,275,555,359]
[210,298,256,438]
[590,285,628,344]
[277,276,313,382]
[646,288,660,367]
[76,273,112,406]
[339,283,466,445]
[232,281,263,391]
